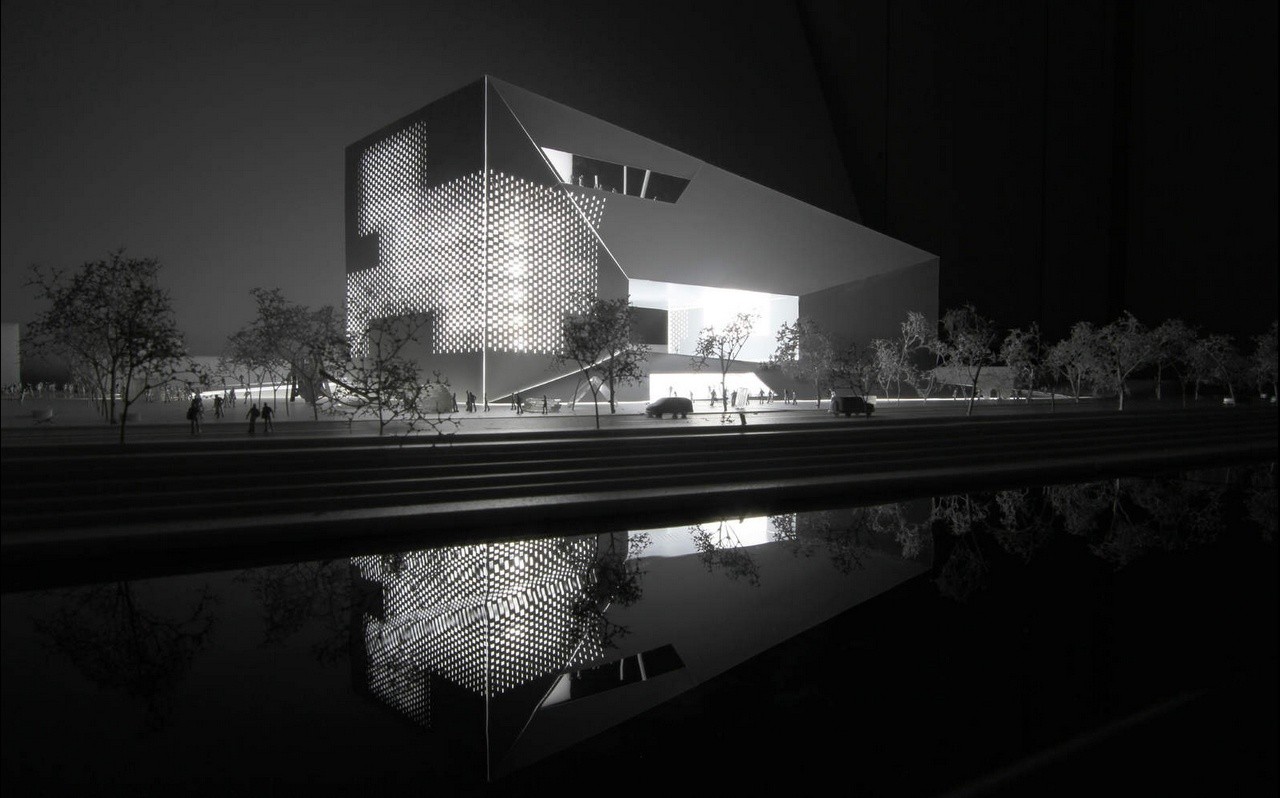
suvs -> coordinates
[646,397,693,419]
[827,396,876,417]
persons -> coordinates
[0,382,275,437]
[452,390,548,415]
[674,388,835,406]
